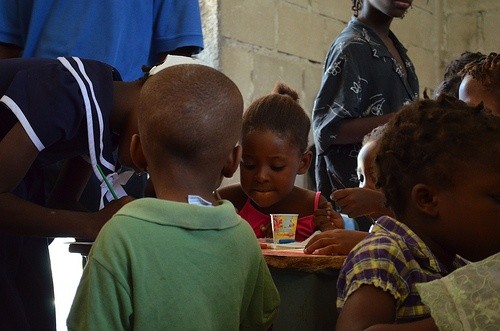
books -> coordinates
[260,229,322,256]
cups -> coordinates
[270,213,298,244]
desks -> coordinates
[69,239,348,331]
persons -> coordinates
[0,0,500,331]
[67,64,282,331]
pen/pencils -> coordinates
[326,167,377,225]
[454,251,476,265]
[215,187,223,206]
[95,163,119,200]
